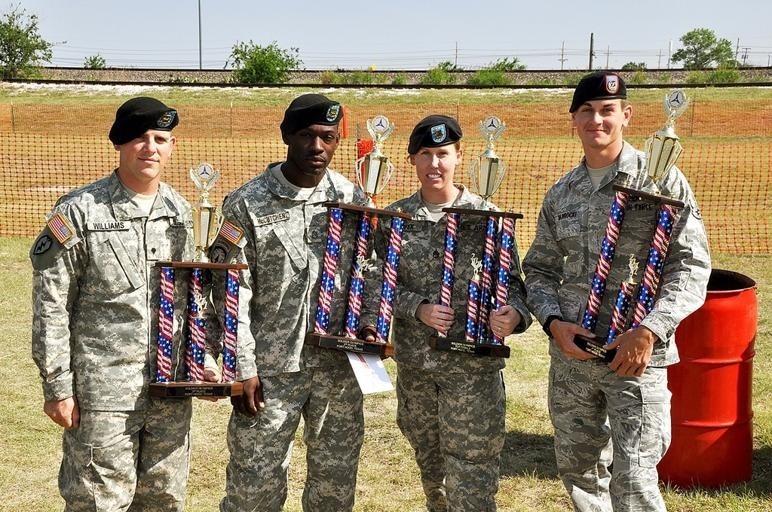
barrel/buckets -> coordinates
[656,269,756,490]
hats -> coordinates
[109,97,179,145]
[569,72,626,113]
[408,115,462,154]
[280,93,344,139]
